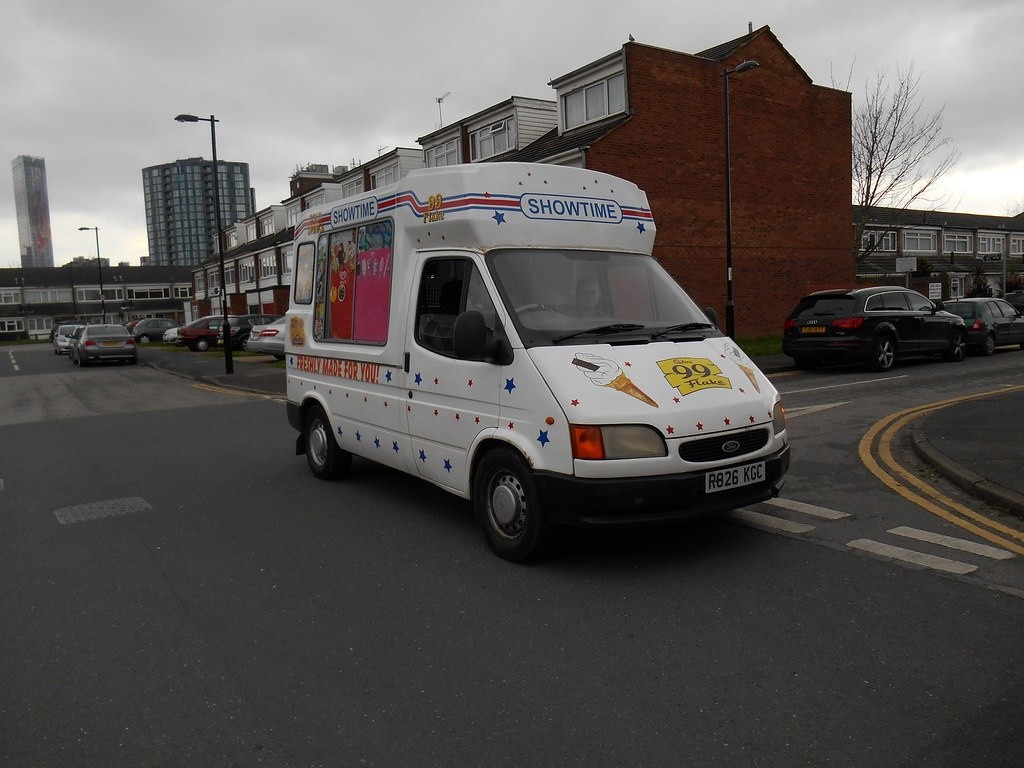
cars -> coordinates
[65,327,85,359]
[247,315,286,361]
[125,321,140,335]
[72,324,138,366]
[163,325,187,347]
[54,325,81,354]
[177,315,239,352]
[133,317,182,344]
[942,297,1024,356]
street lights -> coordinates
[174,113,235,375]
[720,58,760,344]
[79,227,105,325]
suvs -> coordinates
[783,285,969,371]
[50,320,79,341]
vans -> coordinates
[217,313,283,352]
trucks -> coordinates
[285,162,792,563]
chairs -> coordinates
[418,277,463,353]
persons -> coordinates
[49,330,54,343]
[545,274,611,318]
